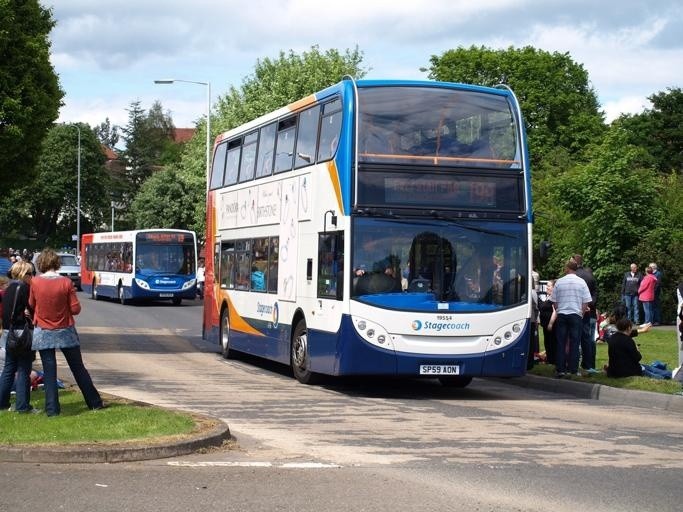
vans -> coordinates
[34,251,80,292]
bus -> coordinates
[80,227,200,308]
[197,74,536,399]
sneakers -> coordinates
[672,367,681,380]
[553,367,599,377]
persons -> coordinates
[0,260,38,413]
[250,265,264,291]
[351,232,394,276]
[28,248,102,416]
[0,246,36,334]
[492,253,672,380]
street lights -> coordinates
[108,197,116,231]
[65,121,88,264]
[152,78,215,214]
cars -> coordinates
[195,262,206,300]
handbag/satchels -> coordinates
[6,321,35,364]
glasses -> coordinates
[25,272,31,276]
